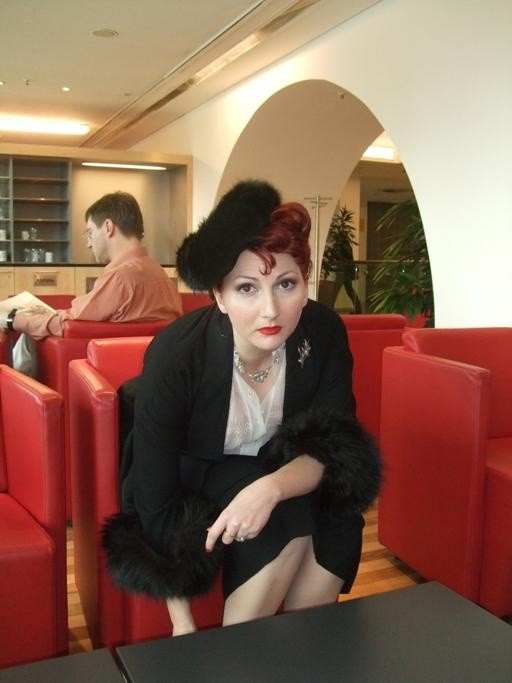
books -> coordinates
[0,290,55,317]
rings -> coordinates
[235,536,245,542]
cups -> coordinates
[21,247,53,262]
[0,229,6,240]
[1,250,8,261]
[21,226,39,240]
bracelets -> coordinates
[7,308,18,333]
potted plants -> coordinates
[315,202,358,313]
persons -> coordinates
[0,189,184,344]
[98,178,386,627]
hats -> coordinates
[177,182,277,286]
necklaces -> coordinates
[233,346,282,385]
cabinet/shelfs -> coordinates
[0,154,75,263]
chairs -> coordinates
[344,314,511,619]
[1,291,227,662]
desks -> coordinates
[1,647,126,682]
[118,579,512,682]
